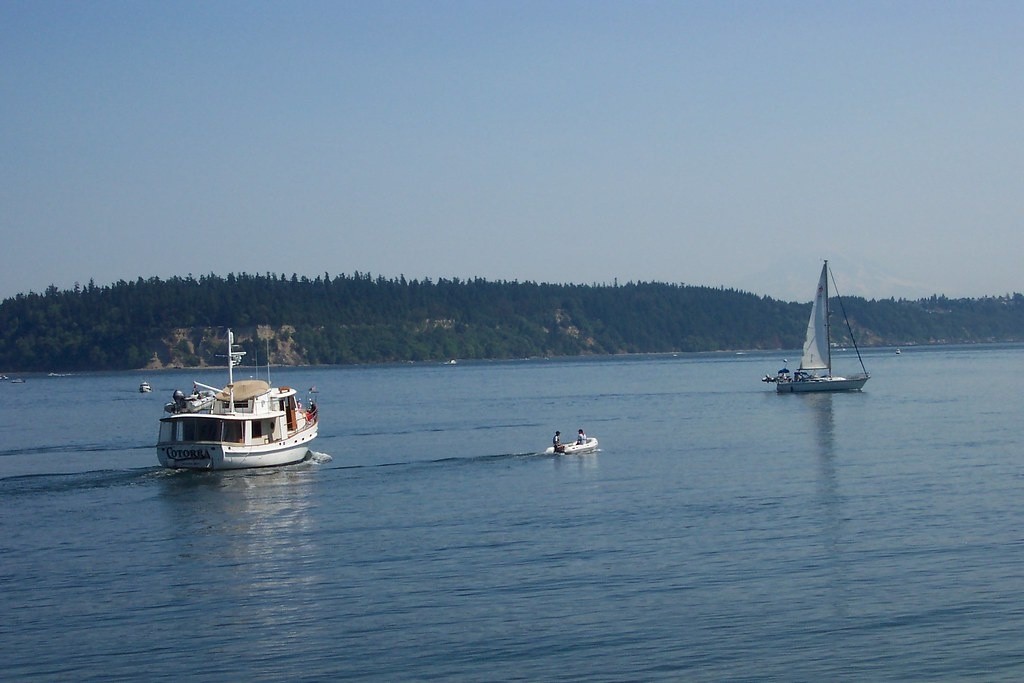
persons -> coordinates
[297,401,316,422]
[576,429,586,445]
[192,385,202,399]
[553,431,560,453]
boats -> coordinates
[836,348,848,353]
[443,359,457,366]
[156,328,318,471]
[735,353,752,358]
[0,375,9,380]
[896,349,901,355]
[48,372,71,377]
[12,378,26,384]
[139,382,152,393]
[546,438,599,455]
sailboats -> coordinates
[761,260,871,394]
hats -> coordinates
[556,431,561,435]
[578,429,583,433]
